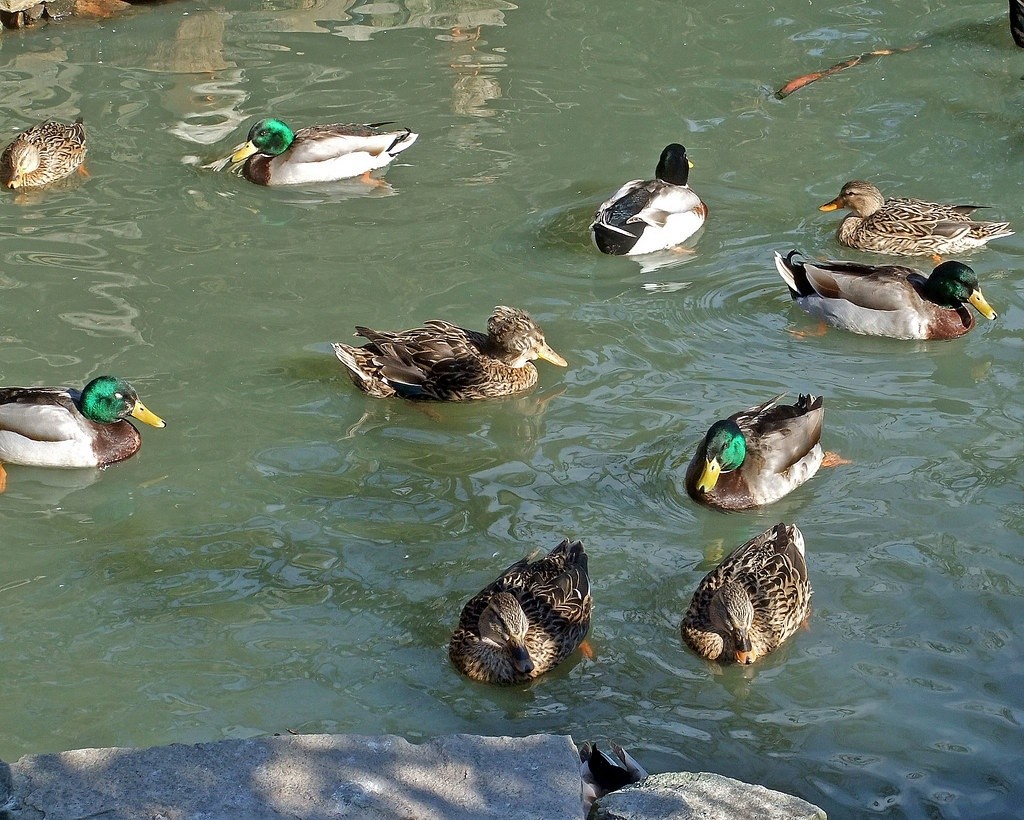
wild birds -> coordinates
[773,250,998,341]
[331,304,567,405]
[229,118,420,188]
[682,523,812,666]
[1,117,89,204]
[0,376,167,492]
[589,143,709,261]
[819,180,1016,257]
[683,390,853,512]
[449,540,593,687]
